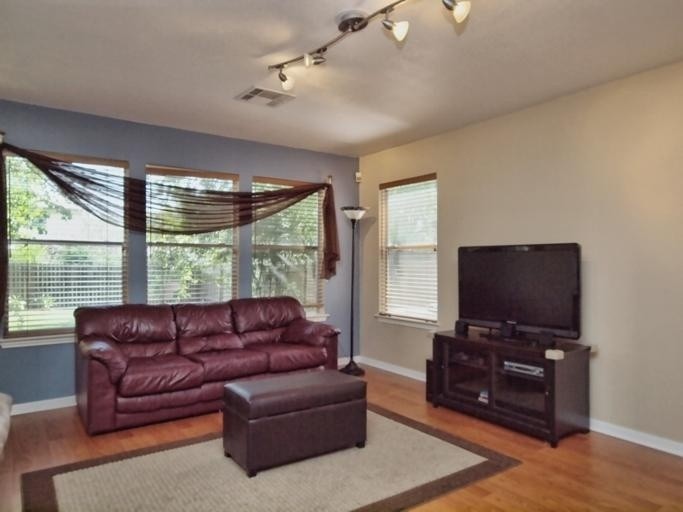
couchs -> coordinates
[73,294,340,437]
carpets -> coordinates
[20,401,521,512]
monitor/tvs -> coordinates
[459,243,583,344]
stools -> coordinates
[222,368,368,477]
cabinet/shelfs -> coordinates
[425,327,591,448]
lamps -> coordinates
[302,50,327,69]
[277,68,295,91]
[441,0,473,26]
[339,205,369,376]
[380,12,410,41]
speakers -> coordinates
[536,332,555,347]
[454,321,467,335]
